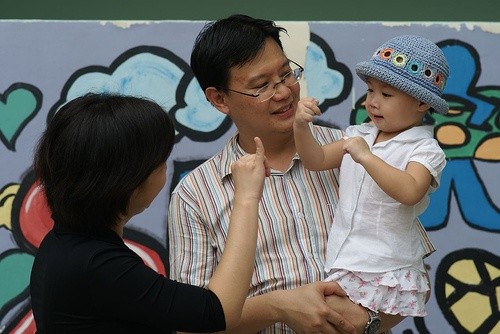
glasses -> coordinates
[225,58,306,103]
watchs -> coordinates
[363,305,384,334]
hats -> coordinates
[354,34,451,115]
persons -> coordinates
[168,12,431,334]
[292,31,453,334]
[29,91,270,334]
